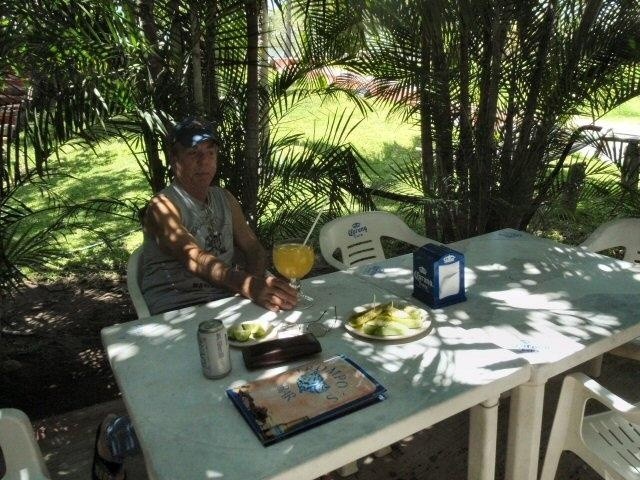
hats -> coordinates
[167,119,222,147]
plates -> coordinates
[344,301,432,340]
[228,319,277,347]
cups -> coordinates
[273,238,315,311]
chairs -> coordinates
[319,211,443,272]
[127,245,151,319]
[540,370,640,480]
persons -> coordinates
[91,122,298,480]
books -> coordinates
[228,353,387,448]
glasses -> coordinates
[282,306,338,337]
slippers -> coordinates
[91,421,128,480]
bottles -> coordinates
[197,318,232,381]
